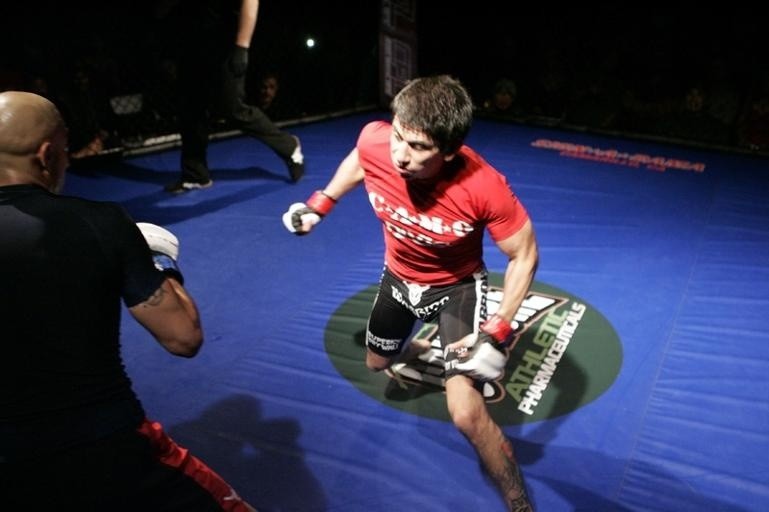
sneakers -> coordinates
[161,178,212,198]
[284,133,306,184]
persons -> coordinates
[163,0,306,195]
[481,79,515,114]
[668,80,769,149]
[282,74,549,512]
[22,74,104,159]
[2,90,253,512]
[248,64,295,121]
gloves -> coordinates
[451,313,515,384]
[134,220,184,284]
[282,189,337,236]
[229,42,249,80]
